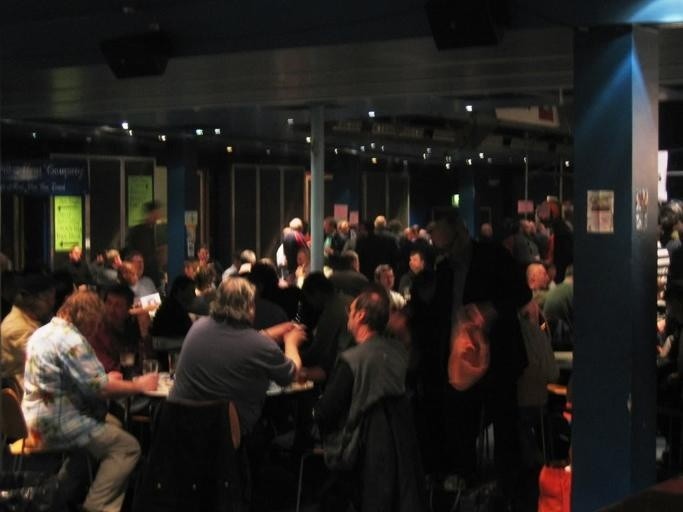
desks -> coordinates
[118,371,314,441]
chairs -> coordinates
[146,395,252,510]
[1,387,98,510]
[295,387,414,512]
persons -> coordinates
[1,198,683,511]
